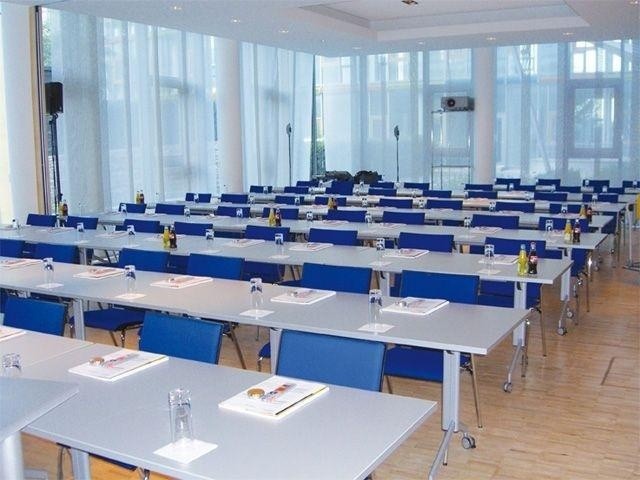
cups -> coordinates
[249,196,256,208]
[168,388,195,447]
[2,352,22,380]
[362,198,368,210]
[483,244,496,273]
[505,179,598,193]
[184,206,191,221]
[368,288,382,328]
[77,223,86,244]
[411,190,417,199]
[592,194,599,207]
[308,177,402,198]
[560,206,569,218]
[127,225,137,248]
[193,194,199,206]
[462,191,468,200]
[463,216,471,234]
[275,234,285,254]
[524,192,531,201]
[205,229,216,251]
[546,219,553,237]
[263,187,268,196]
[235,208,243,224]
[376,238,387,264]
[364,213,373,229]
[419,200,424,209]
[294,196,301,208]
[12,219,20,240]
[305,210,314,228]
[488,201,495,213]
[42,258,55,284]
[123,264,135,297]
[249,277,263,307]
[120,204,128,218]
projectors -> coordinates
[442,96,475,111]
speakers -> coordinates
[45,82,63,114]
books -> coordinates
[217,375,330,420]
[382,296,450,315]
[270,288,337,305]
[0,324,25,343]
[68,348,170,383]
[72,267,125,280]
[150,274,213,289]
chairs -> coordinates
[1,175,640,480]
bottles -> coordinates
[580,202,592,223]
[134,190,145,204]
[518,241,537,275]
[328,195,338,210]
[163,223,178,250]
[267,206,282,227]
[563,218,581,243]
[57,200,69,216]
